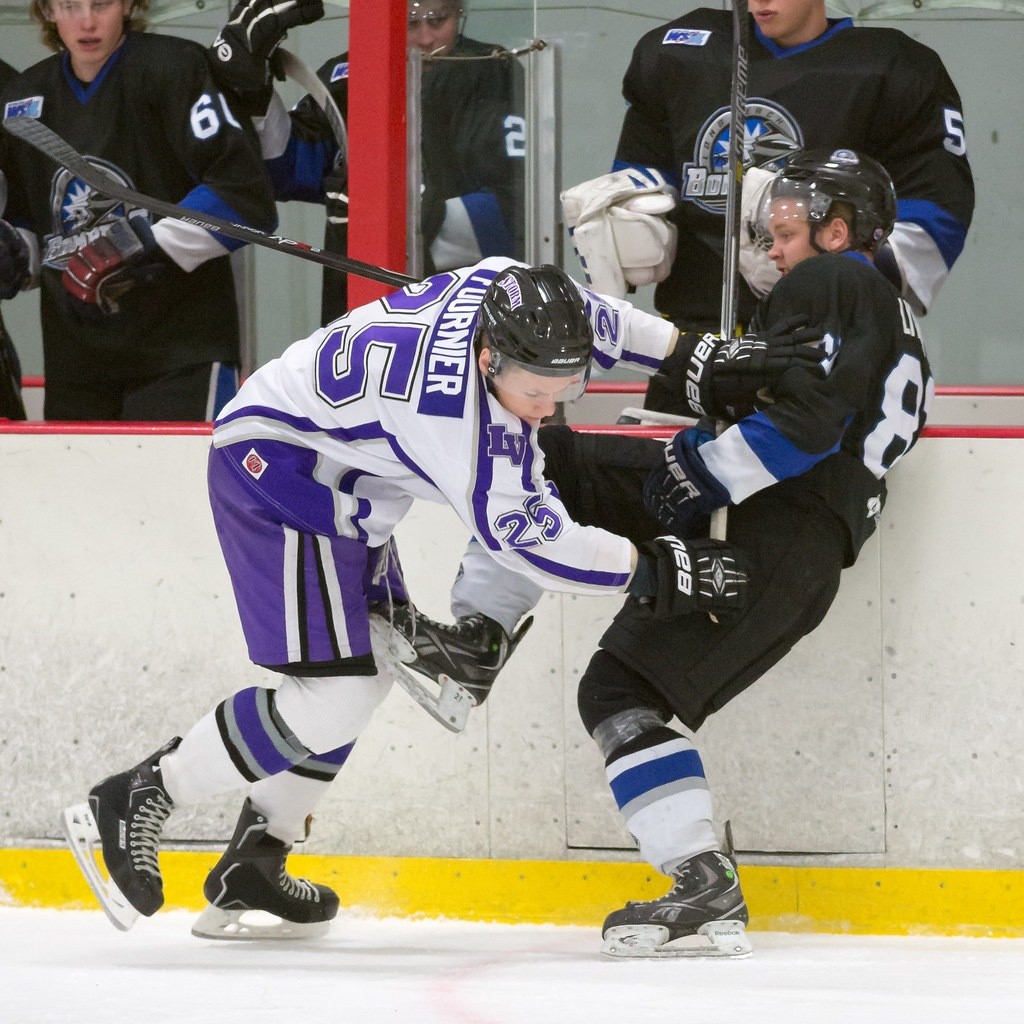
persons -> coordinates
[211,0,525,329]
[561,0,975,418]
[59,257,827,941]
[0,0,279,421]
[396,148,934,957]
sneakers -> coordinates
[63,735,184,931]
[600,819,752,958]
[190,796,341,940]
[364,597,535,734]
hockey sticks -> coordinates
[708,0,750,540]
[1,115,422,288]
[274,46,348,161]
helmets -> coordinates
[772,149,899,249]
[480,262,594,377]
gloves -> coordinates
[0,219,30,301]
[642,429,731,530]
[60,218,172,317]
[682,327,829,422]
[208,0,326,92]
[636,536,754,621]
